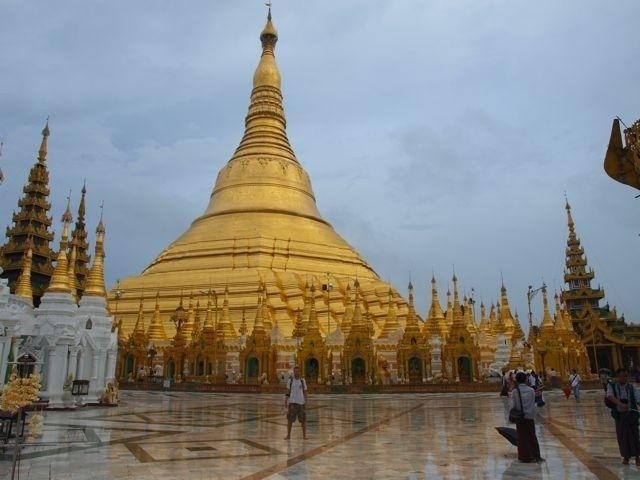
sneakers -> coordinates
[636,458,640,467]
[623,458,628,465]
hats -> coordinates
[571,368,576,371]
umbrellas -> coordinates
[562,383,572,399]
[494,426,519,447]
[599,368,611,371]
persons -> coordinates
[509,372,546,462]
[601,370,612,393]
[550,368,556,386]
[607,368,640,466]
[284,367,309,440]
[569,369,583,403]
[499,367,545,407]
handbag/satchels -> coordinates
[603,383,619,409]
[509,408,524,423]
